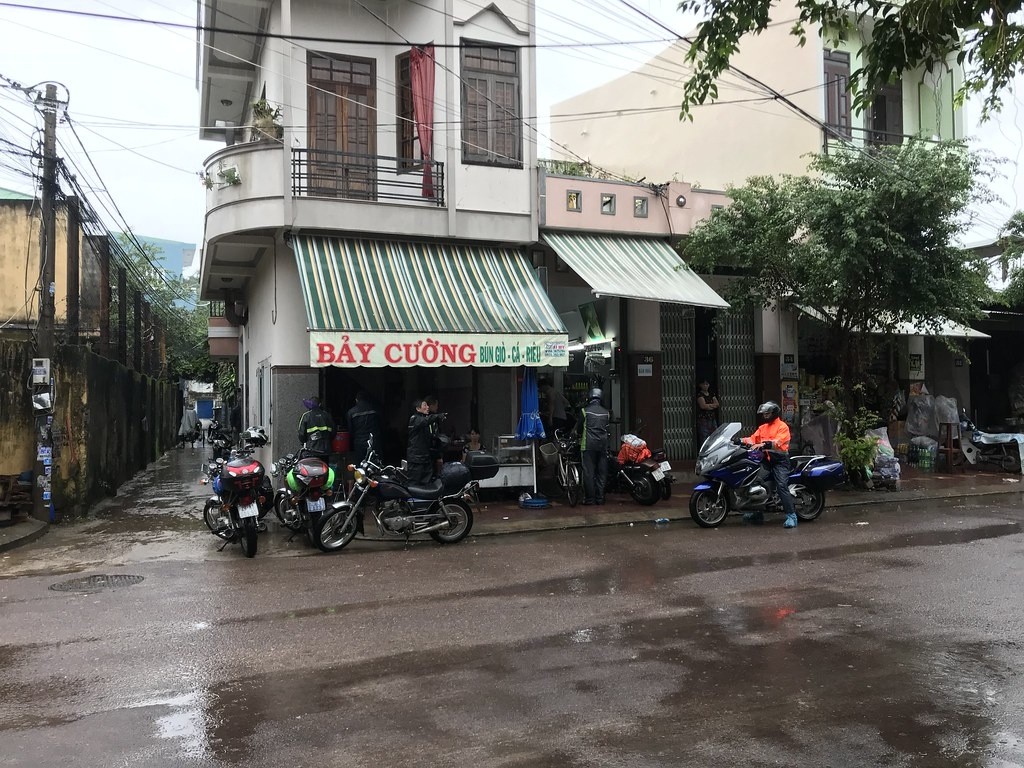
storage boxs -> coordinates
[220,458,265,493]
[465,450,499,480]
[292,458,329,488]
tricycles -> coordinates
[180,421,204,449]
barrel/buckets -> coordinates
[331,432,350,452]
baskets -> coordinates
[539,443,559,464]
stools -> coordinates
[937,423,966,475]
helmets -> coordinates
[761,400,781,422]
[241,426,268,445]
[587,388,604,402]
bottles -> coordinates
[896,443,936,469]
[655,518,670,524]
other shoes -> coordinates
[783,513,797,527]
[742,511,765,523]
[594,498,605,505]
[579,499,594,505]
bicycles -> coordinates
[538,429,581,508]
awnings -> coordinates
[792,302,991,338]
[289,232,570,367]
[539,231,732,309]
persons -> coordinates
[541,378,571,440]
[732,400,798,528]
[696,377,720,448]
[179,403,202,437]
[348,391,381,463]
[462,428,488,502]
[572,388,611,507]
[298,397,338,466]
[407,398,448,487]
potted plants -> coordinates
[253,101,282,139]
[835,432,880,490]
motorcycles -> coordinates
[952,407,1021,474]
[315,433,499,553]
[603,418,678,505]
[208,434,233,463]
[200,425,276,558]
[207,419,224,443]
[269,443,337,548]
[689,421,844,528]
